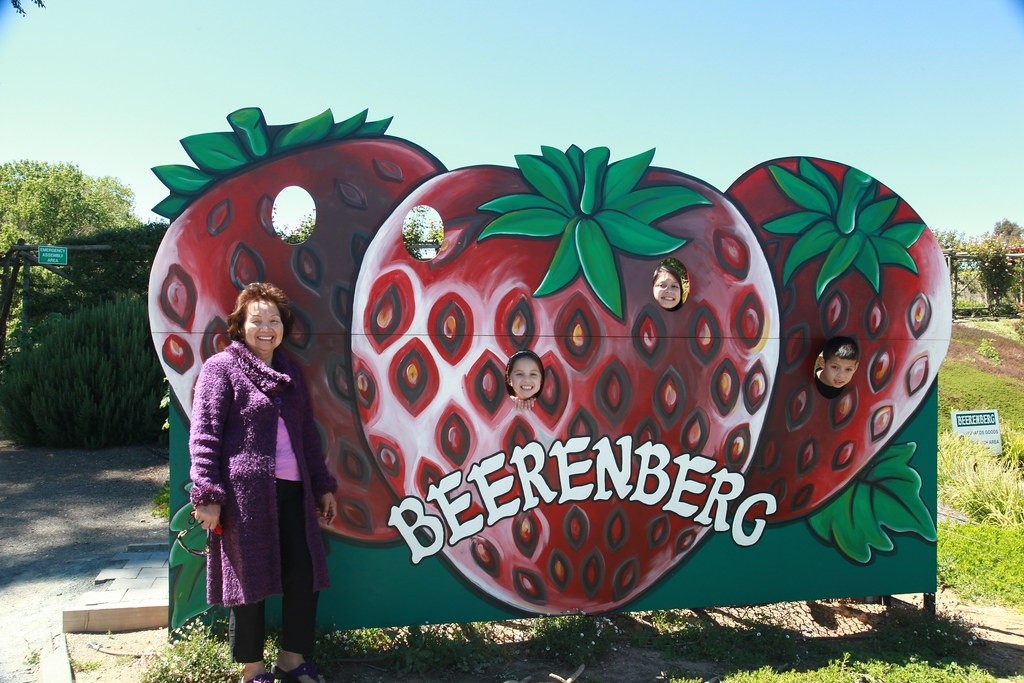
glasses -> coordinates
[653,281,680,291]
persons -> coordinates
[190,281,338,683]
[505,349,546,411]
[651,264,685,312]
[811,334,862,400]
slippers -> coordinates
[242,662,326,683]
